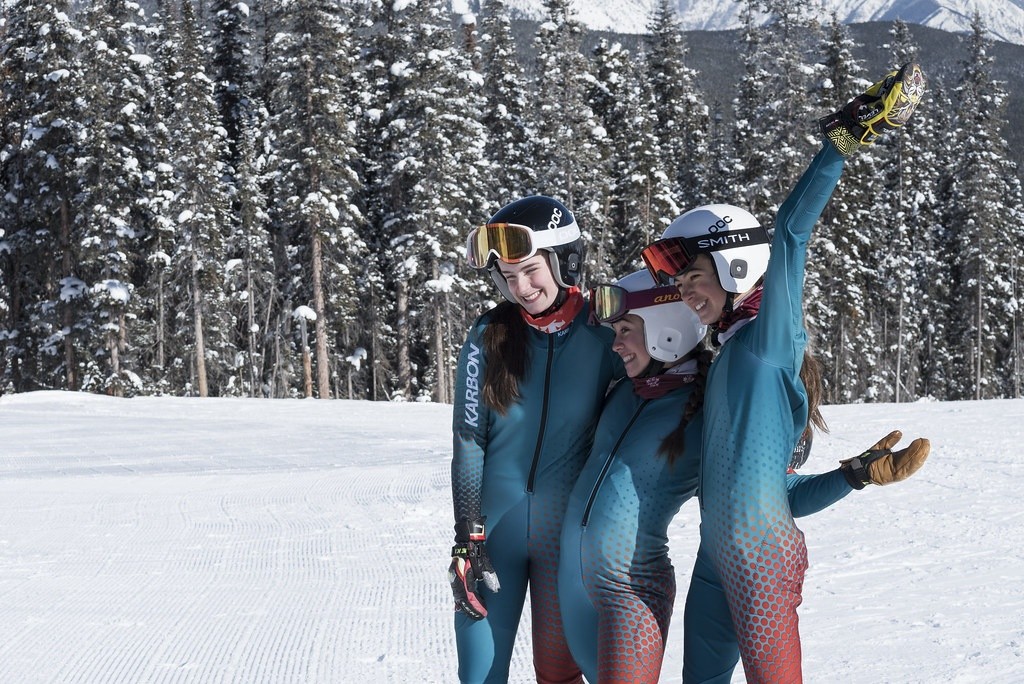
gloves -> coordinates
[820,62,924,157]
[448,516,502,620]
[840,429,930,490]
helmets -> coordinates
[486,196,583,303]
[600,269,707,363]
[659,203,772,294]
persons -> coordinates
[447,195,626,684]
[556,270,931,684]
[640,63,925,684]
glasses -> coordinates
[641,237,697,286]
[587,284,629,326]
[466,222,537,269]
